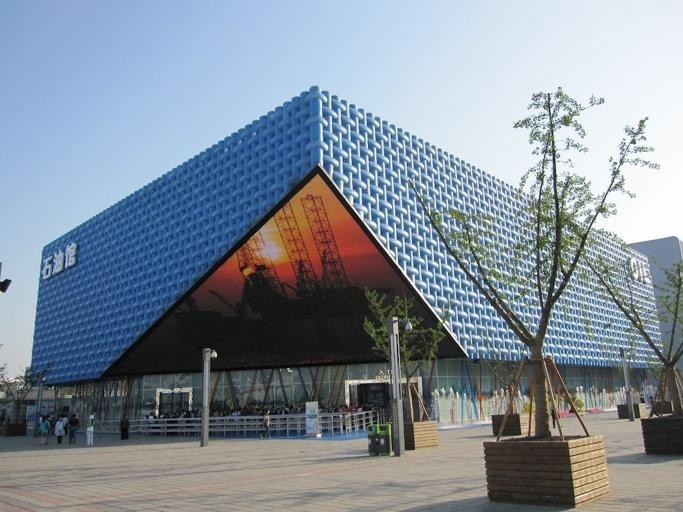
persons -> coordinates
[119,413,130,440]
[145,398,378,440]
[85,414,96,446]
[640,393,646,403]
[650,396,659,416]
[552,393,561,428]
[37,411,80,448]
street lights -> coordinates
[33,374,47,438]
[619,347,637,422]
[387,315,414,458]
[200,347,218,447]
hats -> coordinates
[90,415,94,419]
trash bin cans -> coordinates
[379,423,393,456]
[367,424,378,457]
